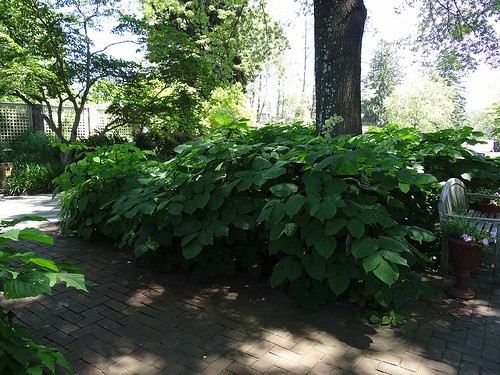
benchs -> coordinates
[440,176,500,266]
